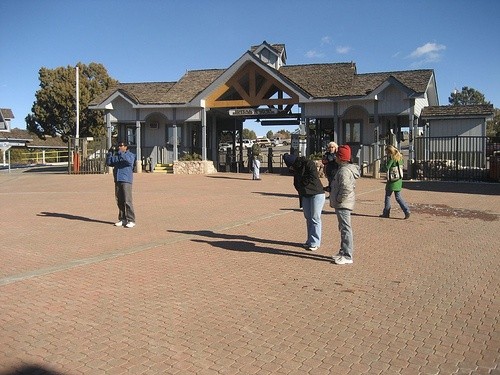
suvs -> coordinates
[254,138,272,148]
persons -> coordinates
[106,140,136,228]
[379,145,411,219]
[330,145,360,264]
[251,139,262,180]
[282,153,326,251]
[323,142,339,199]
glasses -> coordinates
[118,145,125,147]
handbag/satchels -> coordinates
[386,160,403,182]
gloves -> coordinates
[108,148,120,154]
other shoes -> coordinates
[379,214,390,218]
[404,211,411,219]
[253,178,262,181]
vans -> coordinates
[236,139,253,151]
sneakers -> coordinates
[114,221,126,226]
[125,222,135,228]
[303,243,321,251]
[332,254,354,265]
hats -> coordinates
[337,145,352,162]
[282,153,297,168]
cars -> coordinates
[494,151,500,163]
[227,141,245,151]
[271,139,279,148]
[219,143,232,153]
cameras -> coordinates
[114,144,119,150]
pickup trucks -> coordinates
[275,137,292,146]
